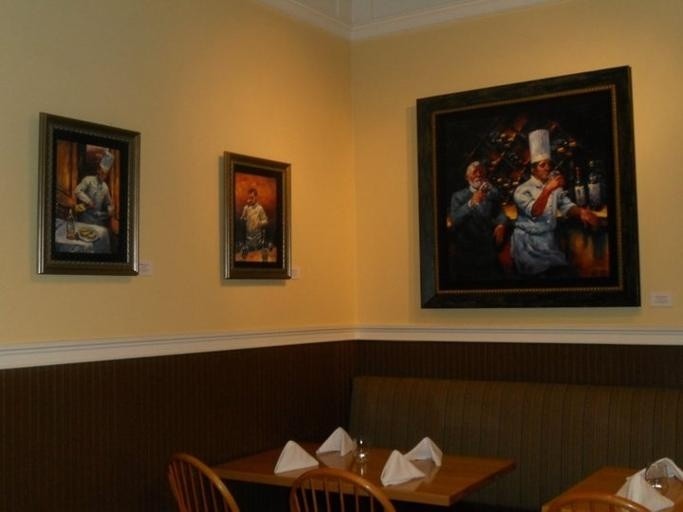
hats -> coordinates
[528,129,552,165]
[100,151,115,174]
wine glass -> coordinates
[549,169,569,200]
[471,181,491,209]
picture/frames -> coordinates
[416,65,642,308]
[224,150,292,280]
[38,111,141,276]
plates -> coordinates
[78,227,100,242]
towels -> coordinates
[616,471,676,509]
[379,448,423,485]
[314,427,355,454]
[272,441,320,477]
[647,456,683,484]
[404,435,445,466]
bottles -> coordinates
[66,208,75,240]
[574,167,588,208]
[587,160,604,211]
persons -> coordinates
[74,161,115,244]
[240,189,268,251]
[510,159,596,286]
[449,160,508,283]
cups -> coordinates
[351,437,371,464]
[645,462,670,496]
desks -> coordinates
[540,465,683,511]
[212,442,514,512]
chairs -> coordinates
[540,491,648,512]
[167,453,240,512]
[289,467,395,512]
